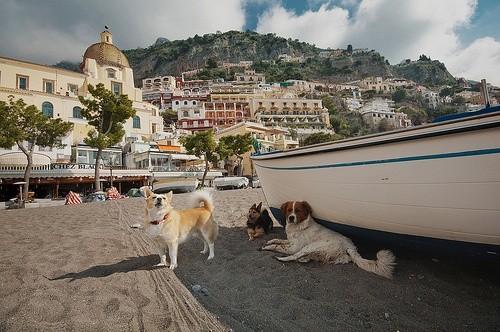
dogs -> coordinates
[143,186,219,270]
[246,202,273,241]
[255,201,399,280]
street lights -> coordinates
[108,151,113,188]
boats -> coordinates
[214,176,249,191]
[249,79,500,264]
[152,177,199,192]
